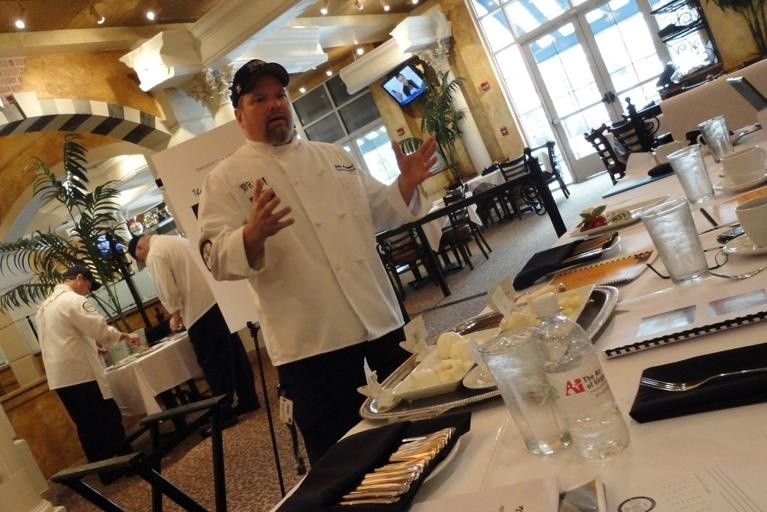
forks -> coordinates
[637,367,766,393]
[707,262,766,280]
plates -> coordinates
[568,192,670,240]
[132,342,164,360]
[723,233,766,258]
[421,438,460,485]
[392,326,500,399]
[714,173,766,196]
[103,358,134,372]
[461,364,496,390]
[495,283,595,396]
[172,331,187,341]
[544,235,621,278]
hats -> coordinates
[231,59,289,107]
[128,235,143,258]
[67,266,101,290]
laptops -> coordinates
[726,76,767,112]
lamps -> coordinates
[321,0,417,14]
[14,2,165,30]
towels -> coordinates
[277,405,474,510]
[626,335,767,425]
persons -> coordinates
[392,73,419,96]
[196,59,439,468]
[128,232,261,438]
[33,264,142,486]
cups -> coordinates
[733,197,766,250]
[722,144,766,186]
[662,144,713,205]
[637,196,710,283]
[695,113,735,164]
[478,332,571,454]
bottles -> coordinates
[531,293,631,460]
[128,219,143,237]
[141,207,166,230]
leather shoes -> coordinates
[205,415,237,435]
[234,401,259,414]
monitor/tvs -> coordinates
[380,63,430,108]
[94,232,123,257]
